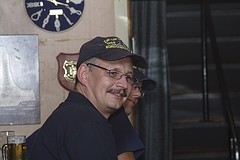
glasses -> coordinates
[132,84,146,98]
[87,63,139,85]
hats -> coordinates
[76,36,146,68]
[133,67,157,92]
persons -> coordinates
[28,36,148,160]
[26,67,158,160]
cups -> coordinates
[2,135,27,160]
[0,131,15,150]
